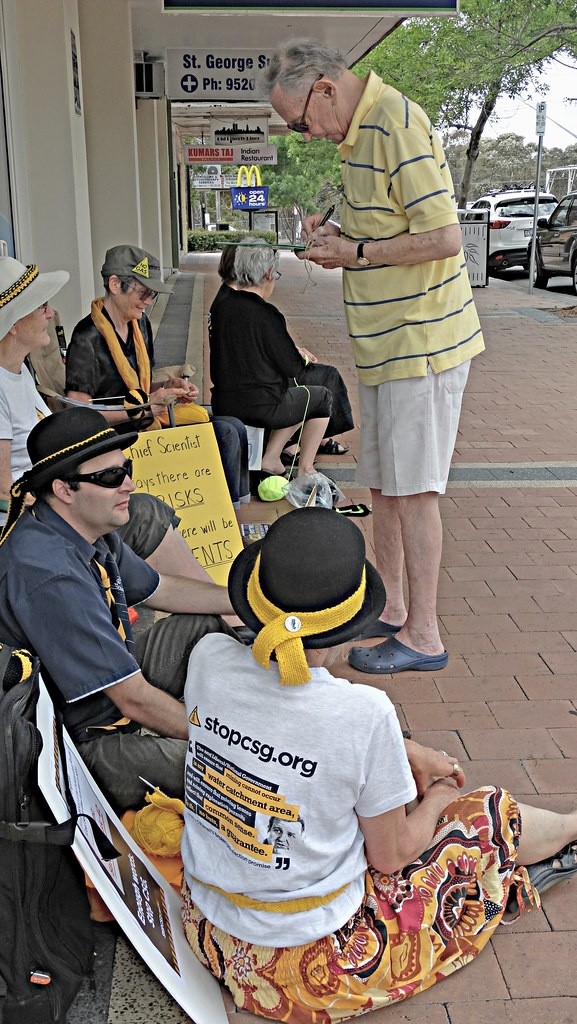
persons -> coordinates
[0,256,70,500]
[254,36,486,674]
[0,407,412,812]
[205,235,356,495]
[180,504,577,1024]
[65,245,260,549]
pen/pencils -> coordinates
[319,205,335,226]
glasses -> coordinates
[40,302,48,312]
[275,270,283,280]
[121,280,158,299]
[67,460,133,488]
[286,74,323,132]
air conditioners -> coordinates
[202,165,221,175]
[135,62,166,99]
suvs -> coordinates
[527,190,577,296]
[469,182,559,275]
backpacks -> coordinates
[0,644,121,1024]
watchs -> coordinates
[356,241,370,268]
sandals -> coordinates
[318,437,348,454]
[283,450,317,466]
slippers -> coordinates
[348,619,401,640]
[260,467,296,483]
[348,635,449,674]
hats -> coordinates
[101,246,168,292]
[228,509,387,686]
[2,257,69,341]
[0,408,136,549]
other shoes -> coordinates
[515,842,577,904]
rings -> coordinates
[450,762,459,773]
[440,750,448,757]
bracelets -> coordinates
[164,379,169,388]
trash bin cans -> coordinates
[457,208,490,288]
[216,223,229,231]
